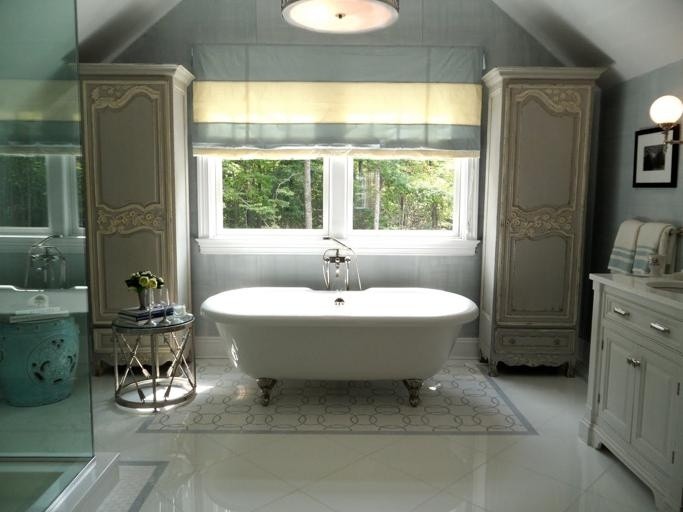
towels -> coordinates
[608,219,677,277]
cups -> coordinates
[172,304,187,319]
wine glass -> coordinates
[143,284,169,325]
[648,254,665,277]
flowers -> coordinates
[125,270,163,288]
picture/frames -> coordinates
[632,124,680,187]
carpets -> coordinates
[134,357,538,435]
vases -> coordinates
[138,289,154,310]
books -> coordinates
[111,304,173,326]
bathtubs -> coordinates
[200,284,480,379]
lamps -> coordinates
[279,1,398,35]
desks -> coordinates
[112,312,197,408]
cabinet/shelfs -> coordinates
[579,280,683,511]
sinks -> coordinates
[647,281,683,295]
[0,285,88,314]
